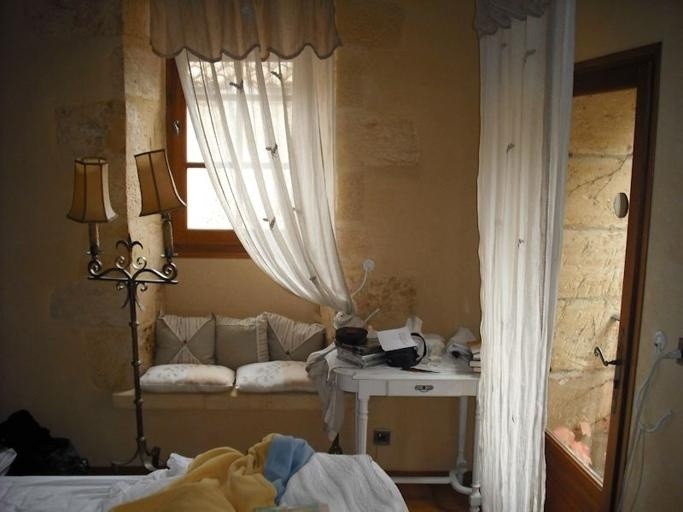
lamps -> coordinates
[66,147,188,474]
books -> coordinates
[468,342,481,373]
[336,335,387,368]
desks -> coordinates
[323,350,483,512]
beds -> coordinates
[0,449,408,512]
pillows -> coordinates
[141,311,325,393]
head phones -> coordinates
[385,332,427,368]
